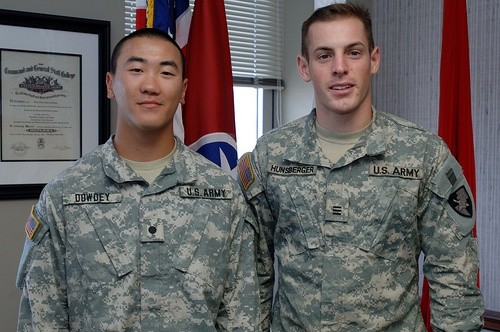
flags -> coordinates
[136,0,238,181]
[420,0,481,332]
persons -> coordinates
[15,28,262,332]
[237,1,486,332]
